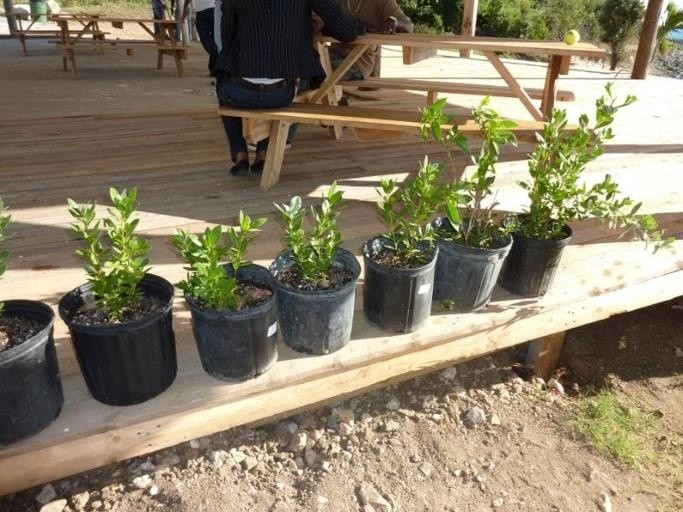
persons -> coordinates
[210,0,359,174]
[308,0,415,128]
[152,0,171,41]
[170,0,196,49]
[180,0,223,77]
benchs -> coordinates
[333,78,575,117]
[215,105,600,192]
[46,38,190,80]
[9,30,112,57]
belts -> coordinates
[230,77,285,92]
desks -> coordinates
[0,13,99,31]
[304,33,607,141]
[47,17,178,46]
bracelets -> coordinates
[389,16,397,28]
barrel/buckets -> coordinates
[29,1,48,23]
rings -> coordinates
[389,29,392,31]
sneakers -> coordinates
[251,160,264,173]
[230,160,249,175]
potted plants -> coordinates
[0,202,63,448]
[58,186,177,406]
[498,83,673,311]
[361,156,440,338]
[268,181,361,357]
[415,96,513,312]
[168,202,278,382]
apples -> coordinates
[564,29,580,45]
